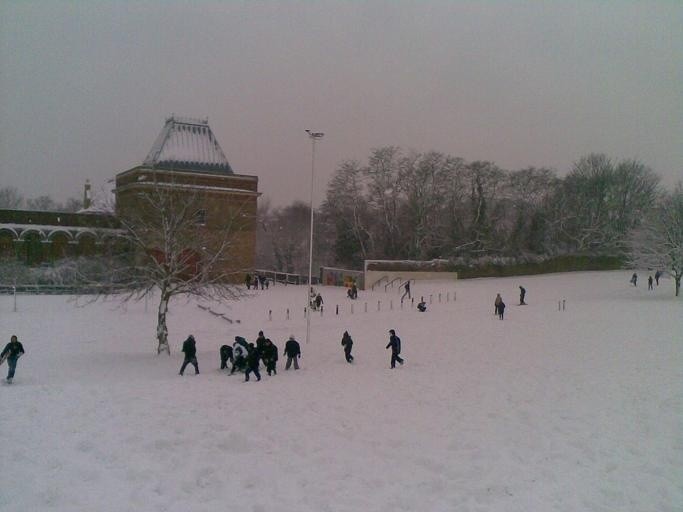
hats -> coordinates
[343,331,349,336]
[289,334,295,339]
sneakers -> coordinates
[7,377,13,384]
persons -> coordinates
[310,286,315,305]
[494,293,501,313]
[178,334,200,377]
[401,280,411,301]
[630,272,638,286]
[0,335,24,384]
[498,298,505,320]
[339,330,354,364]
[350,282,358,298]
[385,329,404,369]
[417,302,427,312]
[314,292,324,311]
[346,288,351,297]
[518,286,526,304]
[217,329,278,383]
[244,273,269,290]
[654,270,661,286]
[282,334,301,369]
[647,276,653,291]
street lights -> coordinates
[304,128,324,308]
